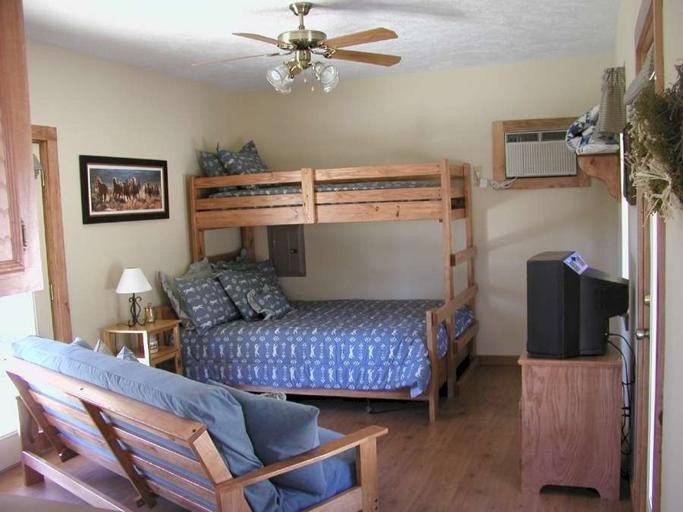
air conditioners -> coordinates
[502,130,576,179]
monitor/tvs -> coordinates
[527,252,629,360]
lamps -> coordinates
[265,49,339,94]
[114,267,151,327]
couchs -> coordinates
[0,336,388,511]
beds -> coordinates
[150,160,479,423]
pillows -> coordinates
[195,140,270,192]
[206,378,326,495]
[157,248,298,336]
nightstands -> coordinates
[102,319,182,375]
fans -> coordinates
[191,4,402,67]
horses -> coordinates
[111,176,162,202]
[95,178,109,203]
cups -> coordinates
[145,306,157,323]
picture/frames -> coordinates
[78,155,169,224]
